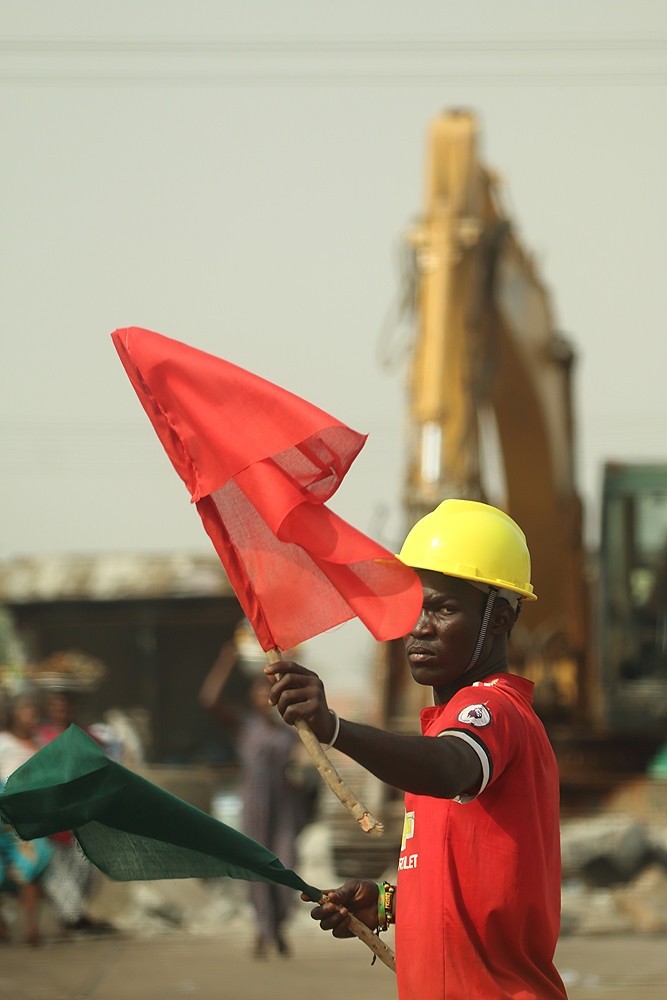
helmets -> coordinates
[370,497,538,608]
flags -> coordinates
[112,326,423,649]
[0,725,321,900]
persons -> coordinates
[202,644,320,961]
[264,499,570,1000]
[0,687,114,946]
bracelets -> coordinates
[323,709,340,751]
[376,881,395,931]
